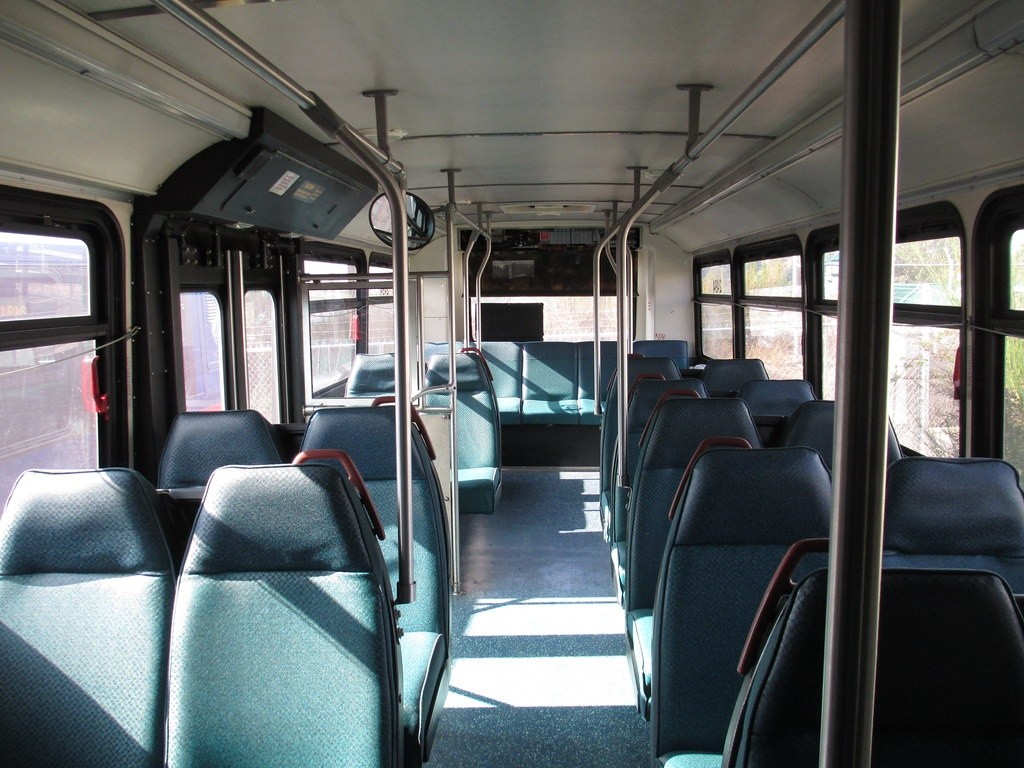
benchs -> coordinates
[0,340,1024,768]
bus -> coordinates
[1,243,221,515]
